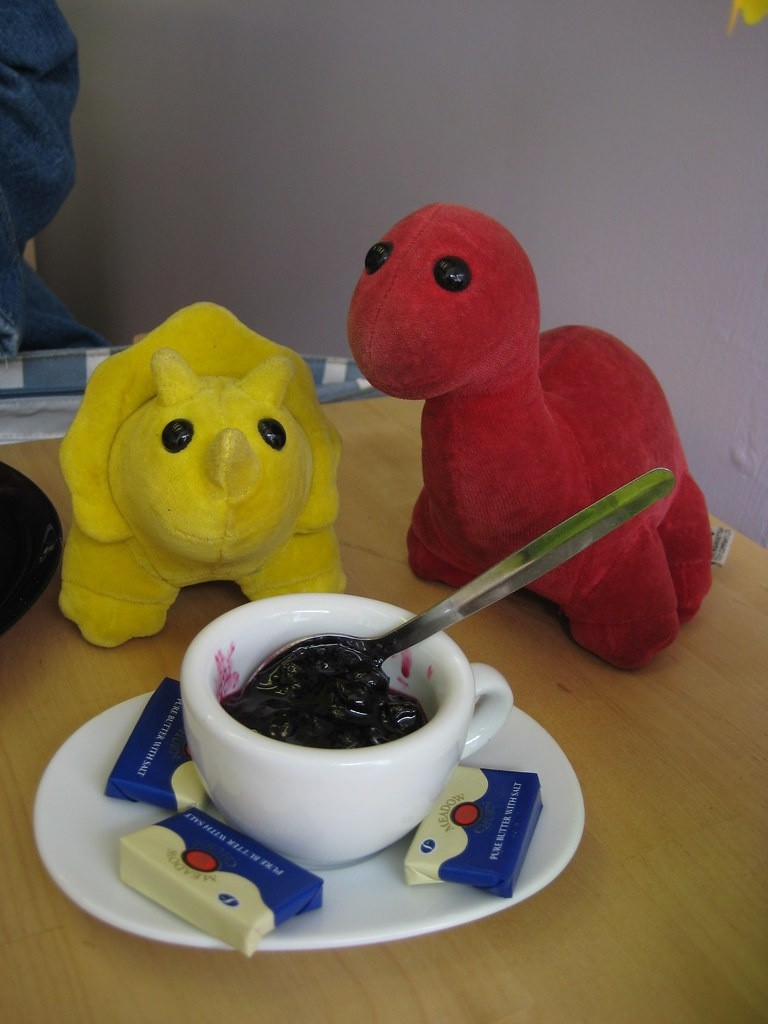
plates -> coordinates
[32,680,585,952]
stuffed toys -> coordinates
[60,302,342,649]
[340,203,712,670]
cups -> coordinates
[180,591,514,869]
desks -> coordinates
[0,399,767,1024]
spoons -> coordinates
[243,468,679,699]
